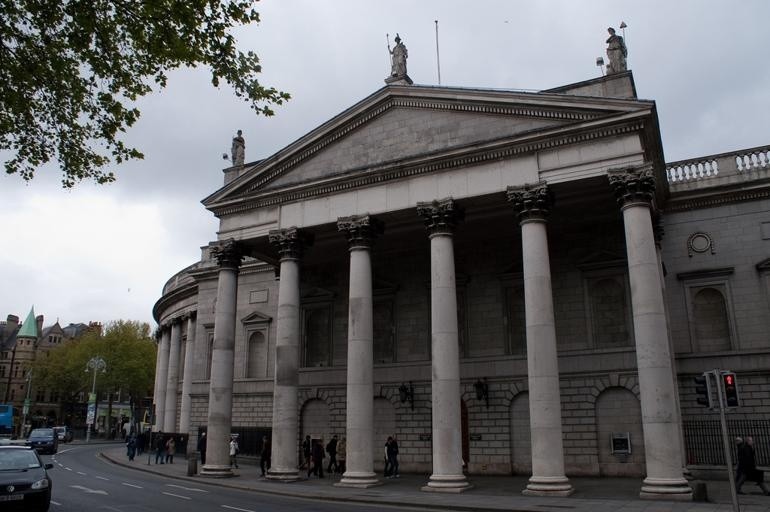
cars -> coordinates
[24,425,74,454]
[0,439,52,510]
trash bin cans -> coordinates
[187,453,198,476]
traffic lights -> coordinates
[722,372,739,409]
[693,374,714,410]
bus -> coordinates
[0,404,50,434]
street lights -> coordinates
[85,353,107,443]
[19,368,33,438]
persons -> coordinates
[228,436,241,469]
[735,436,770,496]
[734,436,763,487]
[387,34,410,76]
[389,438,402,479]
[257,434,272,477]
[122,430,178,466]
[198,431,206,466]
[604,26,629,74]
[382,435,394,480]
[298,432,348,480]
[229,128,248,167]
